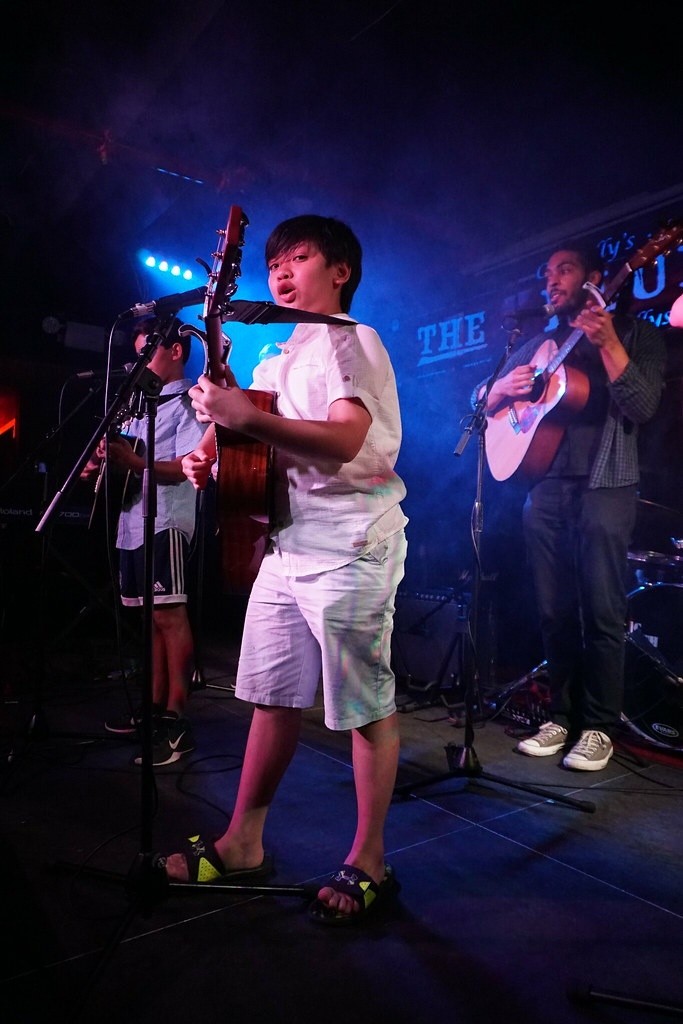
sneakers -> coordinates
[518,721,576,757]
[562,729,614,770]
[133,710,195,766]
[104,700,163,732]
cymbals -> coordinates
[637,498,683,531]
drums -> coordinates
[619,547,683,752]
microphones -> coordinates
[401,627,431,637]
[116,283,210,322]
[68,362,135,381]
[505,303,556,323]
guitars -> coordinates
[197,203,276,597]
[484,217,683,493]
[88,388,146,547]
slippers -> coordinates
[308,859,393,923]
[163,834,264,883]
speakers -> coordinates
[393,584,500,693]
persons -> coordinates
[152,213,409,924]
[470,246,664,771]
[94,316,212,765]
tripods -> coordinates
[32,304,327,1024]
[187,488,235,698]
[392,313,597,815]
[0,372,144,790]
